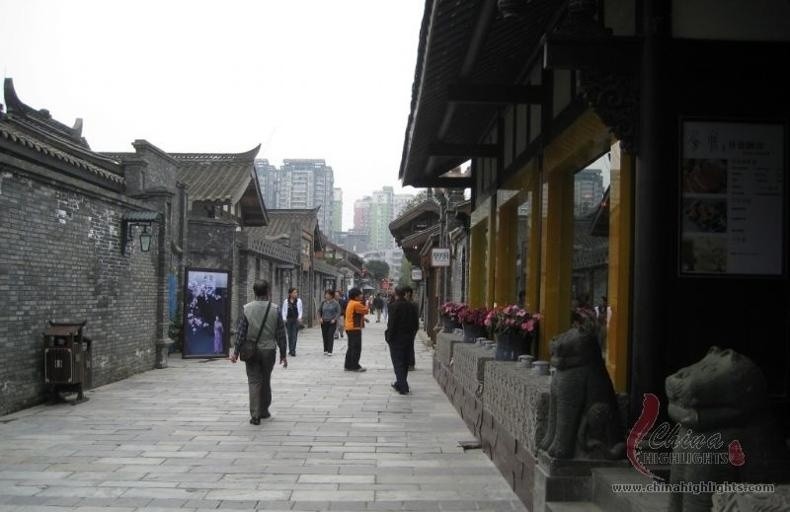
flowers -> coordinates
[440,300,544,335]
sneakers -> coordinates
[344,367,366,372]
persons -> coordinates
[344,288,370,372]
[282,288,303,357]
[403,285,419,370]
[383,285,417,395]
[363,290,398,323]
[316,289,341,356]
[334,291,345,339]
[230,279,287,424]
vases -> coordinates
[494,332,524,362]
[462,324,479,343]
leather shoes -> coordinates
[250,412,270,425]
[391,382,409,394]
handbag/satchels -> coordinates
[240,336,256,361]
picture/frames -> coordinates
[181,266,233,360]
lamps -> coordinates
[125,221,157,252]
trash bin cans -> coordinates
[41,318,92,406]
